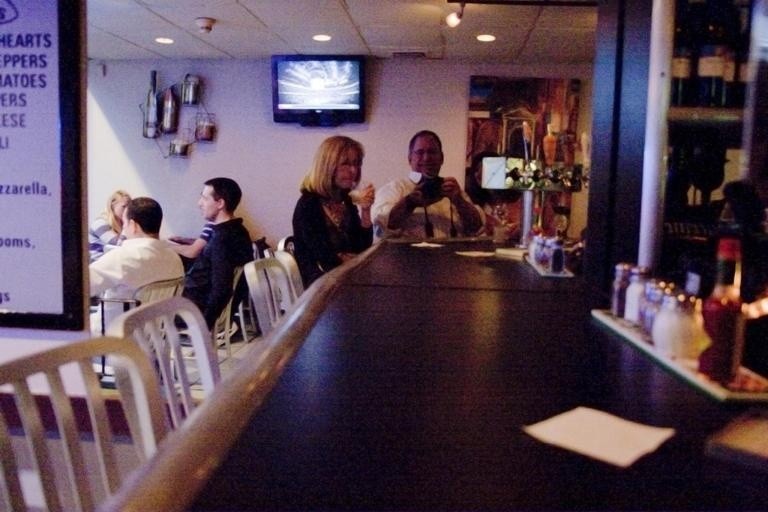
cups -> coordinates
[350,180,370,206]
[170,140,189,156]
[181,81,200,106]
[195,122,215,142]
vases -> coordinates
[514,189,535,249]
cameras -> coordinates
[420,176,444,199]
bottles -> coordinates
[670,1,751,111]
[143,70,160,138]
[161,87,178,133]
[611,263,702,360]
[698,239,744,382]
[528,234,564,274]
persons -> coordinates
[88,190,132,264]
[656,139,768,375]
[168,176,255,335]
[88,196,186,346]
[168,220,215,259]
[370,130,488,239]
[292,135,376,291]
[464,151,524,243]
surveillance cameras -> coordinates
[195,18,216,34]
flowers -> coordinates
[501,153,574,194]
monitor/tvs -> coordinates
[271,55,365,127]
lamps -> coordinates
[444,0,468,28]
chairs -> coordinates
[1,231,307,512]
